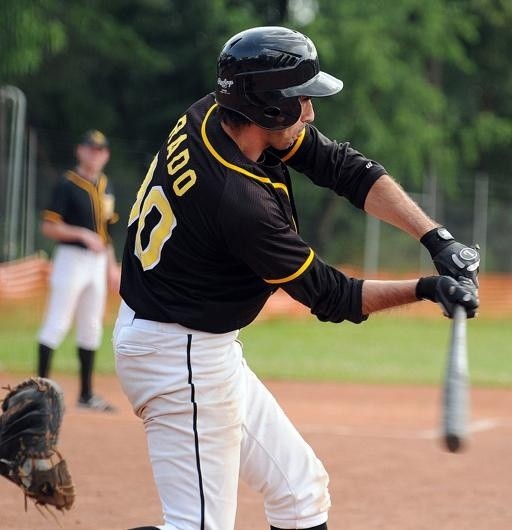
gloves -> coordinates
[416,275,479,319]
[421,224,481,292]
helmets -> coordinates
[214,24,344,130]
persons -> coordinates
[113,25,481,530]
[38,129,120,414]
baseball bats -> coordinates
[445,306,469,453]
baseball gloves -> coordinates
[0,377,74,527]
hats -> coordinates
[75,128,110,150]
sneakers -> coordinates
[77,395,116,414]
